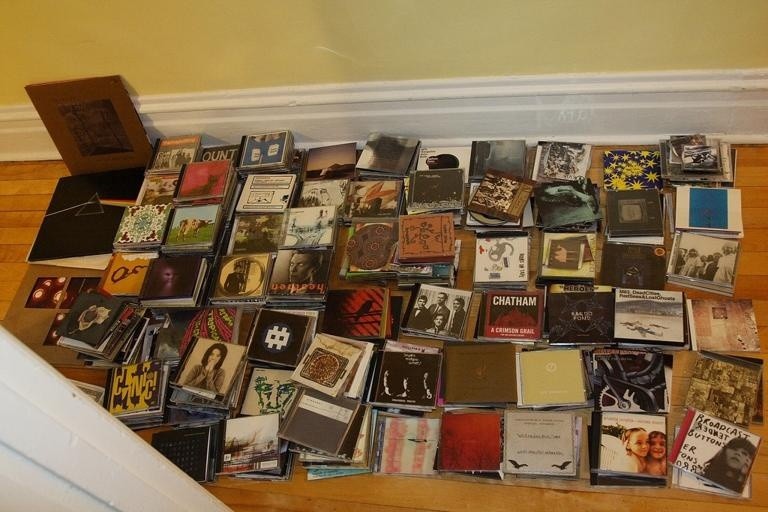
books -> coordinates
[30,134,765,499]
